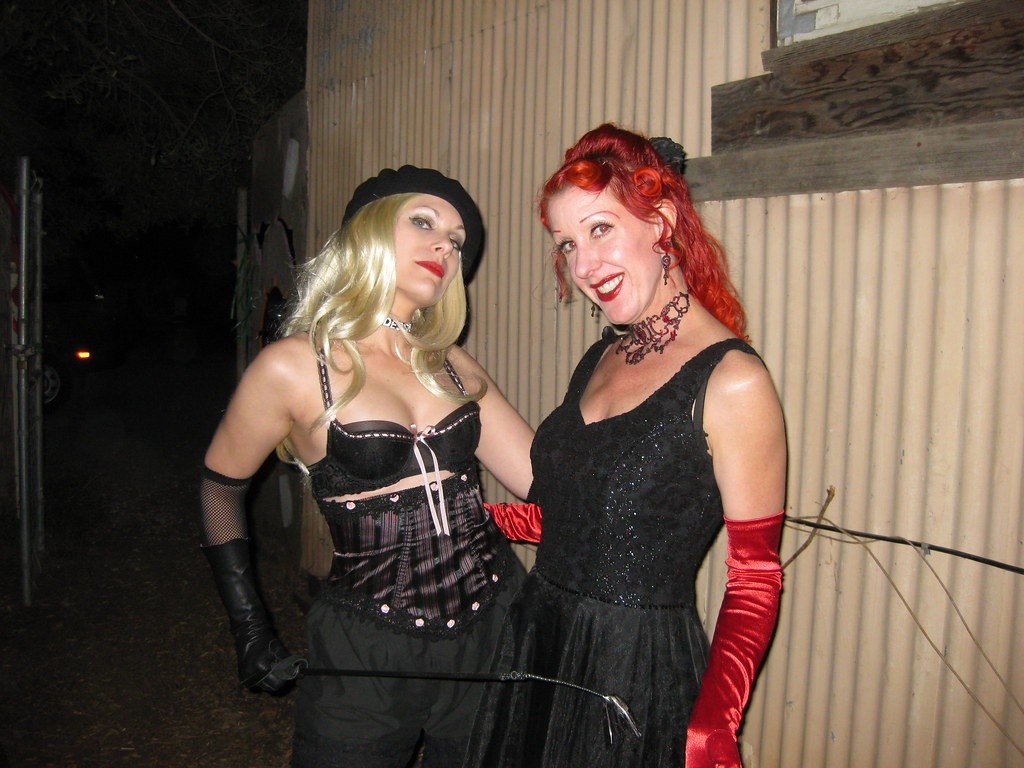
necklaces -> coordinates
[615,285,700,365]
[381,318,414,332]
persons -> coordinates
[201,165,539,768]
[463,124,787,768]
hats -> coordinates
[342,163,485,281]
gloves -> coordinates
[681,510,784,768]
[482,502,542,543]
[198,537,298,697]
